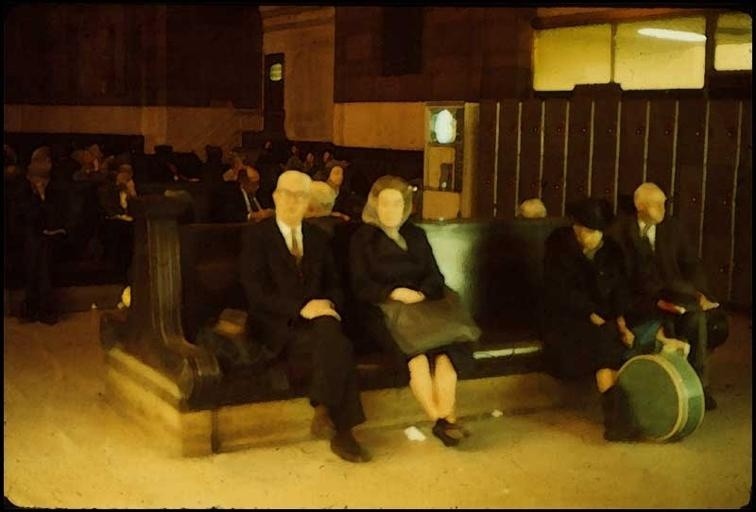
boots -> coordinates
[596,381,644,441]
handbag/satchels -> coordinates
[374,282,482,360]
[189,302,293,377]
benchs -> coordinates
[95,193,728,459]
[1,132,428,328]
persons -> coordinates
[601,178,730,412]
[1,134,364,325]
[235,168,370,464]
[343,173,471,448]
[516,197,546,219]
[541,200,644,444]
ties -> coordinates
[289,228,300,261]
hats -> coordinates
[564,195,620,230]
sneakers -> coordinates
[432,412,474,446]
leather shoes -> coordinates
[329,435,372,463]
[310,412,339,440]
